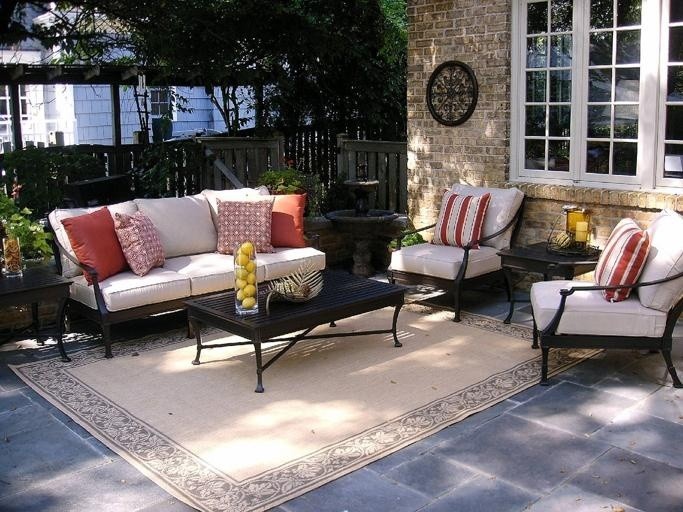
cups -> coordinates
[565,208,592,251]
[233,242,259,316]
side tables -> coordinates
[0,267,74,362]
[494,239,603,327]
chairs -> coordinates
[389,183,526,323]
[529,208,680,389]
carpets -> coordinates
[6,297,604,511]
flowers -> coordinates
[0,184,54,260]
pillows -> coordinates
[430,189,490,251]
[60,205,163,287]
[215,193,304,256]
[593,215,652,303]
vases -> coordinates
[2,238,22,278]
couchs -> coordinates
[47,185,326,360]
